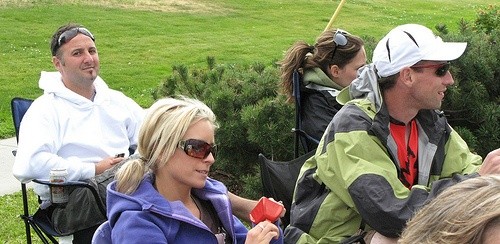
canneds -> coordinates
[49,169,69,203]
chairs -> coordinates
[10,71,320,244]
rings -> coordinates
[258,223,264,229]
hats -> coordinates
[372,24,467,78]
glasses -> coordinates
[411,62,451,78]
[54,27,95,55]
[177,138,218,160]
[329,28,348,68]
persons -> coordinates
[107,95,286,244]
[283,24,500,244]
[12,25,147,244]
[397,173,500,244]
[280,29,367,152]
